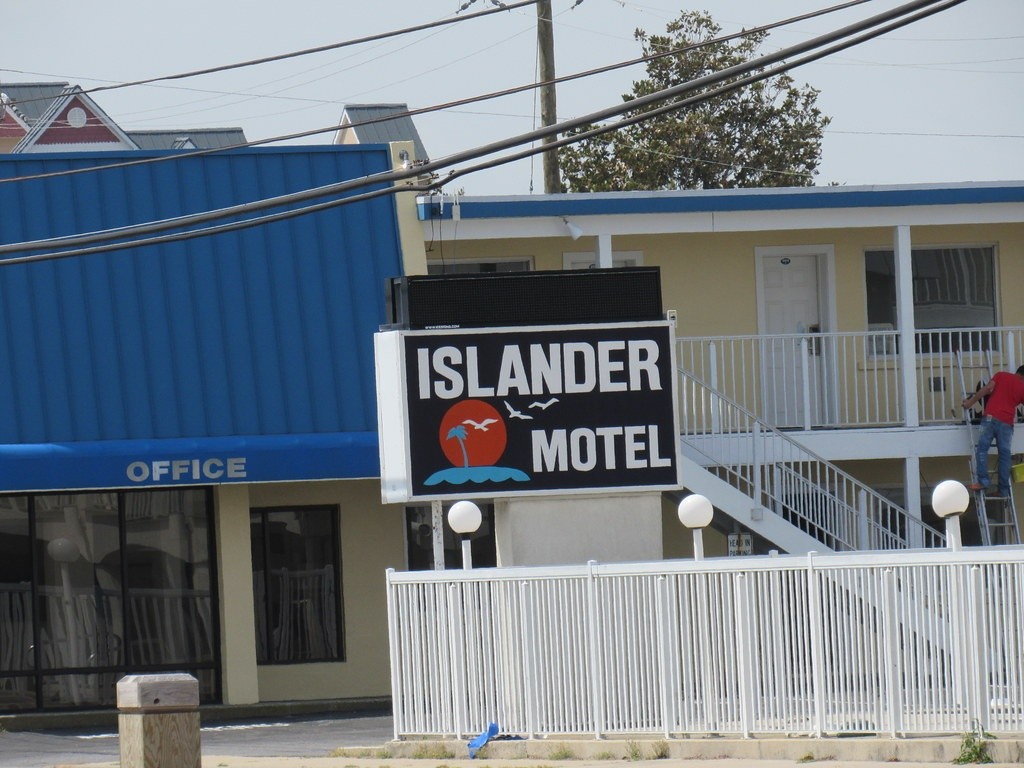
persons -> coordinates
[963,365,1024,499]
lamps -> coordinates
[562,219,583,241]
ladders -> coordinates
[954,347,1022,546]
